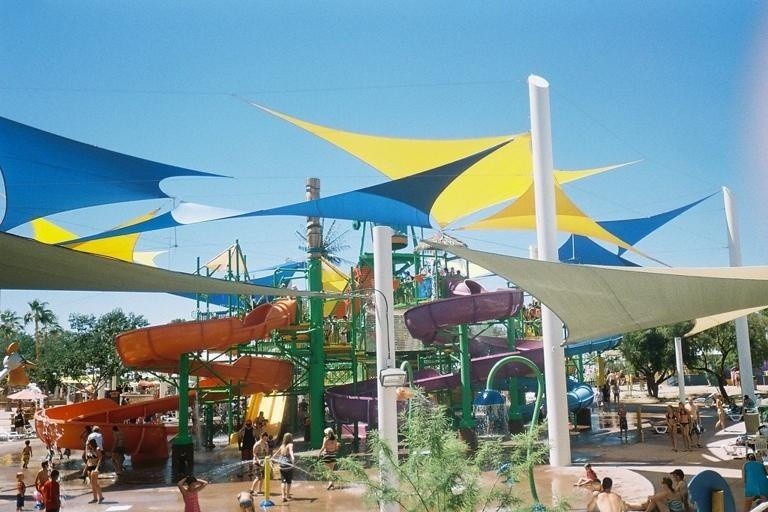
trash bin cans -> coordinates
[743,407,759,434]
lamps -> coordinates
[378,366,407,389]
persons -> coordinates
[403,267,464,292]
[0,352,23,381]
[237,398,341,512]
[117,384,153,424]
[522,301,542,336]
[16,425,125,512]
[6,399,35,439]
[177,477,209,512]
[572,366,768,512]
[324,315,350,344]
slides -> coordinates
[325,277,543,426]
[35,296,301,464]
[474,334,623,435]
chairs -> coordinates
[645,390,767,470]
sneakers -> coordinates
[88,500,97,503]
[98,497,104,503]
